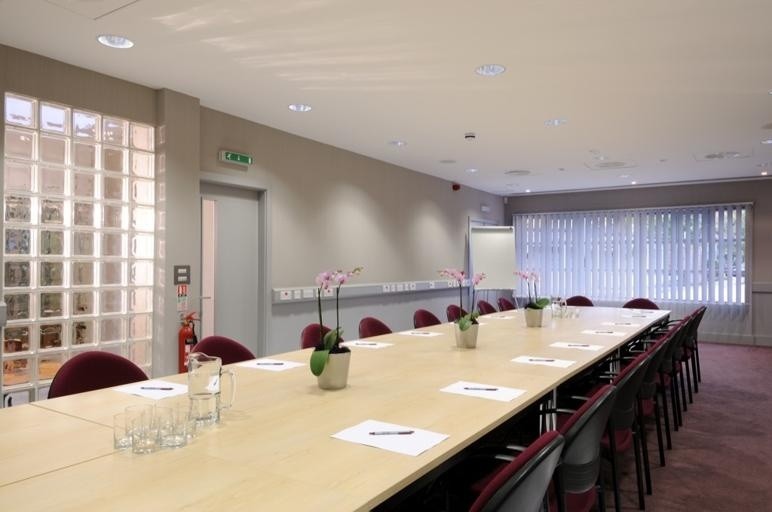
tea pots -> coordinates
[187,351,236,423]
[549,295,567,319]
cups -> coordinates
[563,306,580,318]
[112,396,202,453]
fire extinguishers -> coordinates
[179,312,201,374]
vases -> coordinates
[523,307,542,327]
[314,351,351,391]
[454,324,479,349]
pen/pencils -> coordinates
[529,359,554,362]
[369,432,414,435]
[142,387,172,390]
[356,343,376,345]
[257,363,284,365]
[568,345,589,347]
[595,312,654,333]
[411,332,429,334]
[464,388,497,391]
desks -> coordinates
[2,306,673,510]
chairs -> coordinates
[459,430,565,510]
[413,309,442,329]
[604,307,710,467]
[358,317,393,339]
[497,298,515,312]
[478,299,497,315]
[188,336,256,372]
[506,385,616,509]
[623,298,660,311]
[46,351,150,401]
[302,322,344,353]
[571,352,653,511]
[560,295,594,307]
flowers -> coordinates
[439,265,487,332]
[514,271,550,306]
[310,266,363,375]
